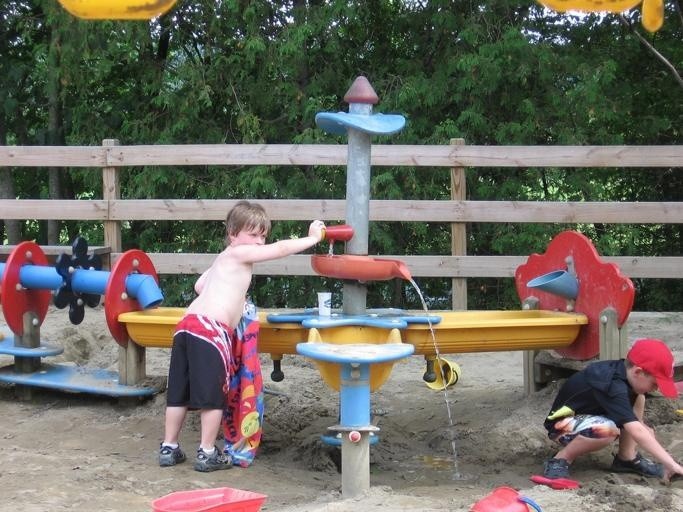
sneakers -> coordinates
[158,441,186,466]
[193,445,234,471]
[544,456,576,479]
[611,451,662,475]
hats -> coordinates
[628,339,679,398]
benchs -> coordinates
[0,245,112,275]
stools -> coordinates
[296,343,414,498]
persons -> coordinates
[158,200,327,473]
[543,339,683,483]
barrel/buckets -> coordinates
[423,358,461,391]
